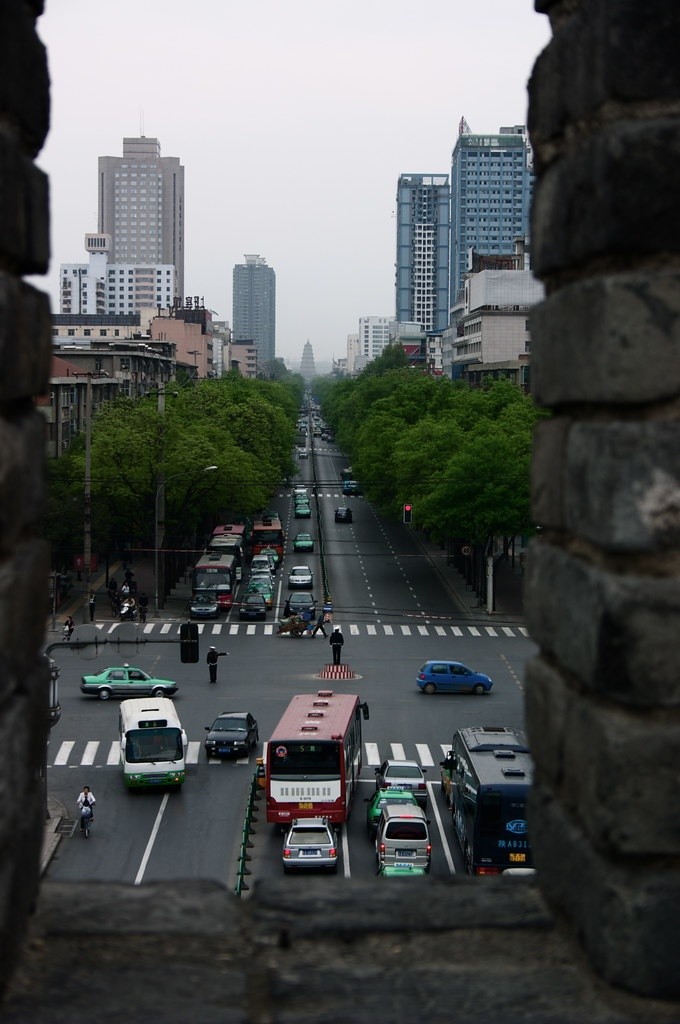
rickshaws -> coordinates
[275,619,331,638]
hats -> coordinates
[210,645,215,649]
[334,626,340,630]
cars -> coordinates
[363,785,419,844]
[416,659,494,696]
[374,758,429,809]
[79,661,179,702]
[204,711,259,758]
[377,864,428,880]
[188,407,364,620]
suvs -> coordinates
[280,818,340,875]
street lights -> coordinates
[152,464,220,619]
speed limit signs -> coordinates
[461,546,472,556]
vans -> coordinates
[375,804,433,873]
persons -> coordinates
[108,578,117,597]
[65,616,74,641]
[76,786,96,832]
[122,582,129,597]
[110,591,120,618]
[125,568,135,583]
[138,593,149,616]
[312,611,329,639]
[89,591,96,621]
[206,646,229,684]
[329,628,344,666]
[283,599,290,619]
[120,597,136,621]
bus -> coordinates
[118,697,188,793]
[437,724,537,876]
[263,689,370,828]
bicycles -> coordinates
[107,578,149,623]
[76,801,94,838]
[60,626,75,642]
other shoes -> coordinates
[90,817,94,821]
[312,635,316,638]
[325,635,329,638]
[80,828,84,832]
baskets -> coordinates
[82,811,92,818]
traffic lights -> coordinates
[402,504,413,524]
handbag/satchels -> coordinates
[83,806,91,816]
